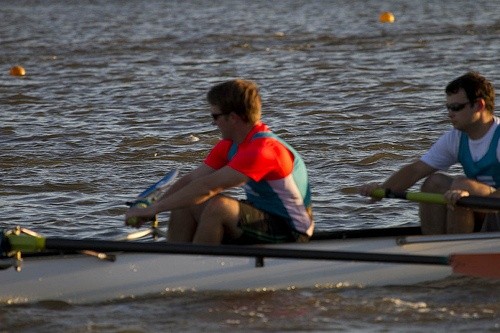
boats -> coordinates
[0,217,499,304]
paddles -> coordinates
[2,230,500,280]
[337,178,500,210]
[124,165,180,228]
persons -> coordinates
[360,73,500,234]
[124,80,315,247]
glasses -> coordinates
[445,101,473,113]
[209,110,230,119]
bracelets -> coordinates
[151,205,155,219]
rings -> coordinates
[364,187,368,190]
